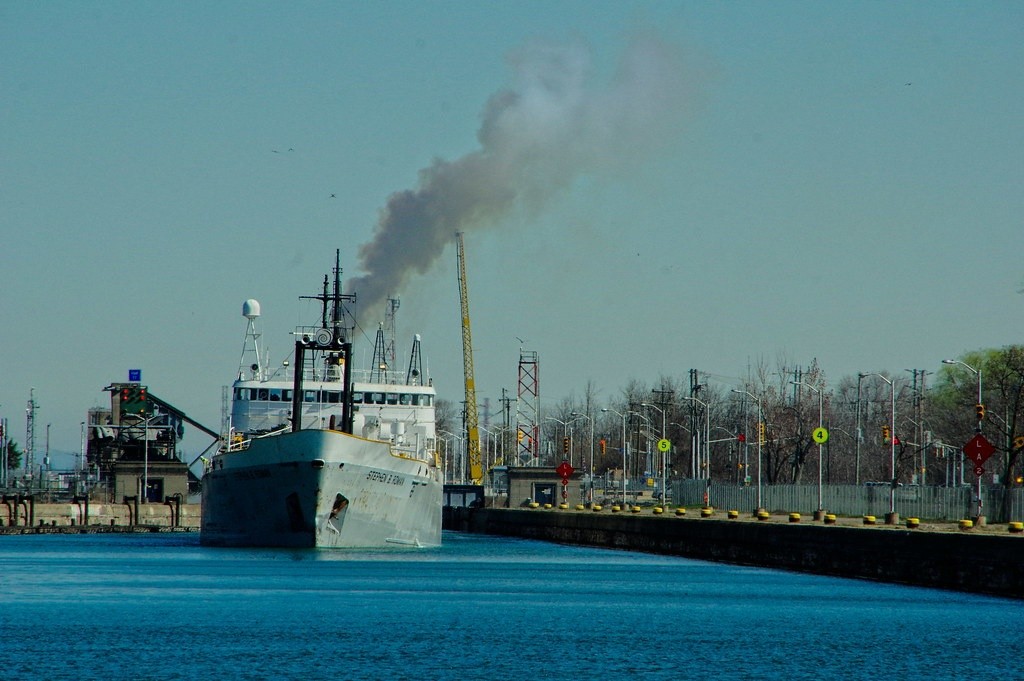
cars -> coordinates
[862,481,922,504]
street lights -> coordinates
[570,411,596,509]
[599,407,629,511]
[434,415,573,505]
[681,396,715,515]
[640,403,670,513]
[858,373,900,525]
[731,389,766,519]
[789,380,827,522]
[941,358,989,527]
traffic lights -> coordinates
[933,445,944,459]
[882,425,889,446]
[976,405,984,421]
[600,440,606,457]
[756,424,765,446]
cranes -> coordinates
[455,228,485,489]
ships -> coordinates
[196,245,446,552]
